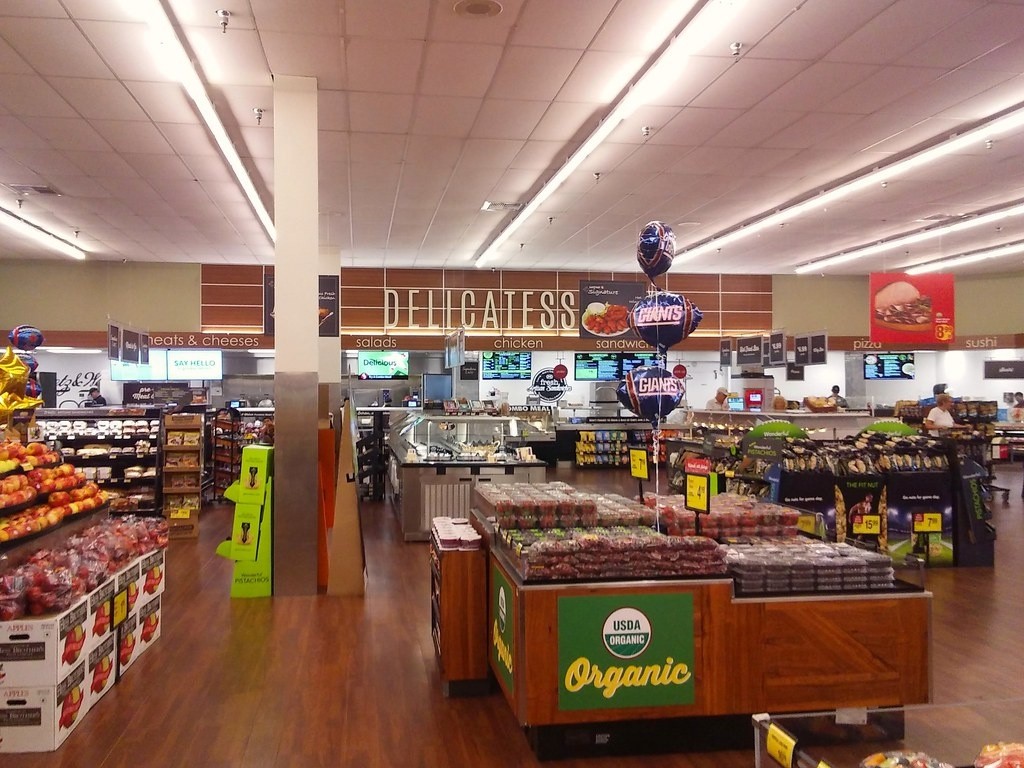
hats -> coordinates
[718,387,730,395]
[89,387,98,396]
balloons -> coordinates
[23,379,42,398]
[613,365,685,429]
[15,352,38,376]
[627,289,703,353]
[0,346,31,398]
[636,218,677,281]
[8,325,44,351]
[0,393,43,415]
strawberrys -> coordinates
[474,480,799,538]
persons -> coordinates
[848,492,872,540]
[263,418,272,427]
[705,387,731,411]
[925,393,968,438]
[1014,392,1024,408]
[88,388,107,406]
[828,384,849,409]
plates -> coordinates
[866,355,877,364]
[902,363,915,374]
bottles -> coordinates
[407,449,415,460]
[239,397,246,408]
[413,392,418,399]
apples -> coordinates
[0,443,108,542]
[0,517,170,621]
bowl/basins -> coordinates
[478,481,656,529]
[633,492,800,537]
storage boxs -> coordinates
[167,516,200,538]
[0,546,167,753]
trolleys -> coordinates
[940,425,1012,502]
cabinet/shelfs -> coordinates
[0,404,275,552]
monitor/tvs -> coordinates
[863,353,915,379]
[482,352,531,380]
[110,349,224,382]
[574,353,667,382]
[358,351,409,380]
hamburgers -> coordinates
[874,281,934,331]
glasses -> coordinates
[947,401,954,405]
